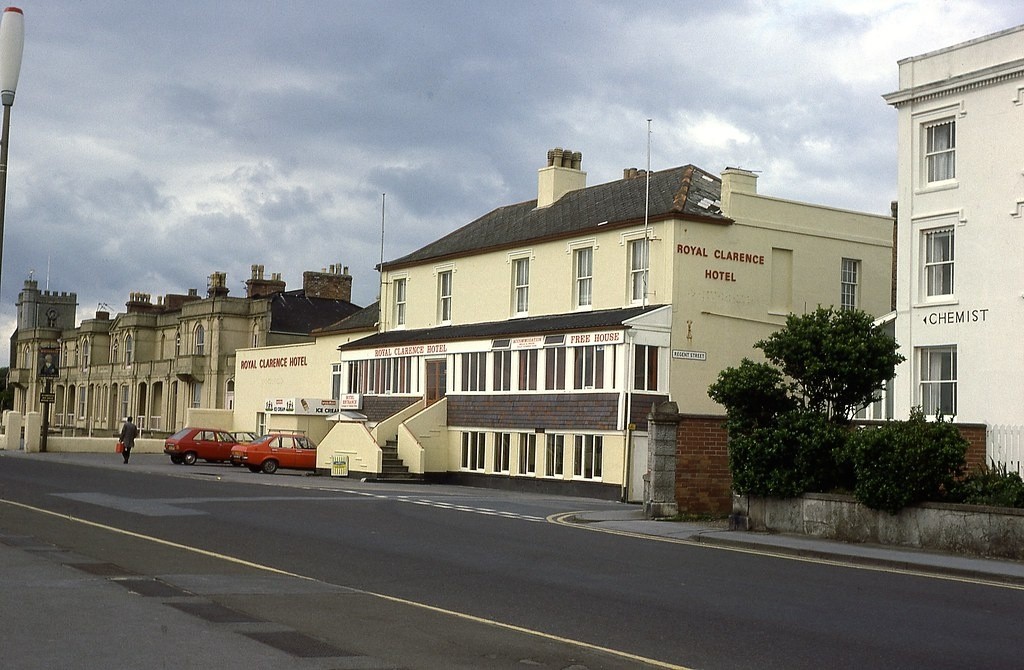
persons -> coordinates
[119,416,137,464]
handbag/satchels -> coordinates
[116,443,123,453]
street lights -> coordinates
[0,5,25,288]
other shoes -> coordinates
[123,458,128,464]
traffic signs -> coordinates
[40,392,56,404]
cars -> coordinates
[211,431,260,446]
[164,427,241,465]
[229,432,318,474]
